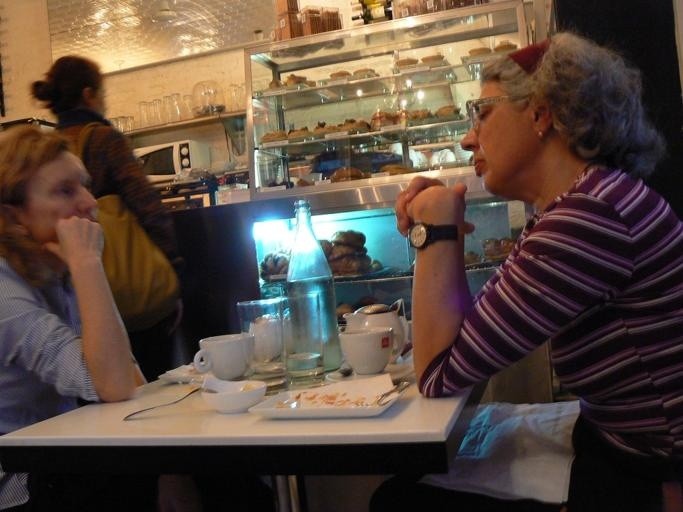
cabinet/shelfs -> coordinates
[243,0,529,203]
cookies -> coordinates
[273,0,341,40]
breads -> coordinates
[269,74,316,87]
[297,179,309,186]
[336,297,376,317]
[382,165,417,176]
[463,238,515,264]
[261,232,382,277]
[331,165,364,182]
[351,0,488,26]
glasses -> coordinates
[467,93,531,129]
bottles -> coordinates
[281,196,347,379]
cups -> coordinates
[105,78,247,135]
[159,291,412,388]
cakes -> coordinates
[397,55,444,67]
[470,44,516,56]
[262,106,461,143]
[331,69,375,79]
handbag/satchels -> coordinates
[94,195,178,333]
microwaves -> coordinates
[131,139,213,188]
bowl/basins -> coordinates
[407,141,475,168]
[198,378,268,414]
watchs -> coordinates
[407,223,458,250]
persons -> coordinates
[369,32,683,512]
[28,55,189,382]
[1,121,203,512]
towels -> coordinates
[415,401,582,507]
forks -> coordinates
[119,386,220,424]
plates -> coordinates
[243,373,409,418]
